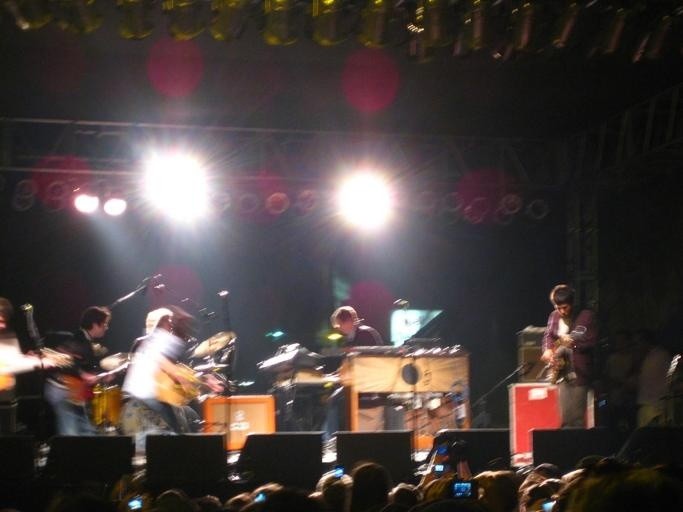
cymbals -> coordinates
[193,363,229,372]
[194,330,236,358]
[99,352,135,370]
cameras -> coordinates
[126,498,142,510]
[448,480,481,500]
[434,465,444,471]
[542,499,557,512]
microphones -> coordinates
[394,298,411,309]
[218,288,231,300]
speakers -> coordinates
[0,433,36,473]
[331,430,413,466]
[45,434,137,470]
[434,430,508,467]
[146,433,226,480]
[532,427,623,474]
[238,431,324,479]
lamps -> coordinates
[1,164,556,231]
[7,1,680,72]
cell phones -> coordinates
[335,467,344,479]
[254,493,265,504]
